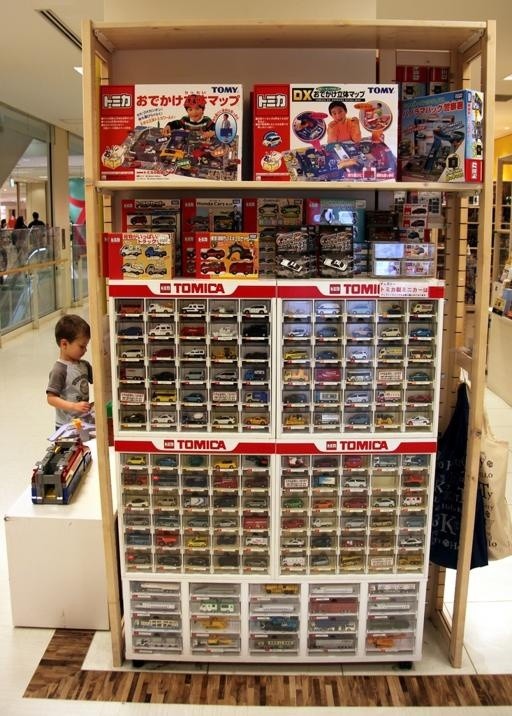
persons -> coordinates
[220,113,231,129]
[45,313,93,434]
[373,102,383,115]
[326,102,361,145]
[0,209,46,277]
[159,94,216,139]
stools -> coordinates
[4,437,119,629]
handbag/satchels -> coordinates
[429,382,512,569]
